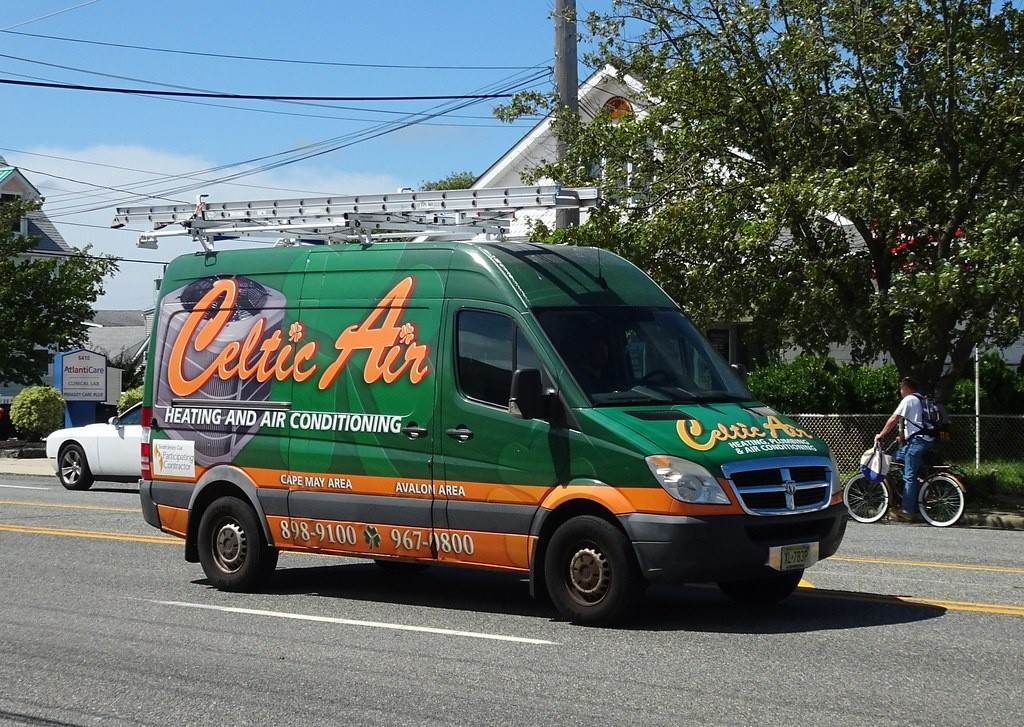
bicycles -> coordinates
[843,436,967,527]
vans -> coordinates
[138,230,848,628]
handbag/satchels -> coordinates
[860,442,892,482]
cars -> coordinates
[45,400,142,490]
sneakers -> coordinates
[890,508,913,523]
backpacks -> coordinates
[912,393,951,436]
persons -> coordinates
[874,377,938,521]
[572,334,653,393]
